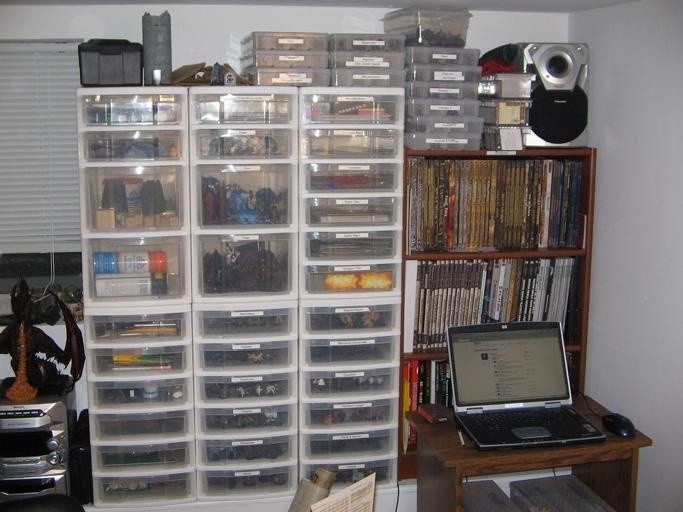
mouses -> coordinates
[602,413,635,438]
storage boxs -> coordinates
[76,87,192,306]
[242,32,407,86]
[83,307,197,508]
[298,299,402,492]
[191,85,299,302]
[380,6,470,46]
[192,302,299,505]
[407,48,486,151]
[299,88,405,299]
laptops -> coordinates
[446,320,607,452]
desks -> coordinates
[405,395,651,512]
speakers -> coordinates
[479,42,589,147]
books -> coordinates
[403,257,576,354]
[401,352,452,455]
[404,156,583,257]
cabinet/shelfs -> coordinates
[397,149,595,483]
